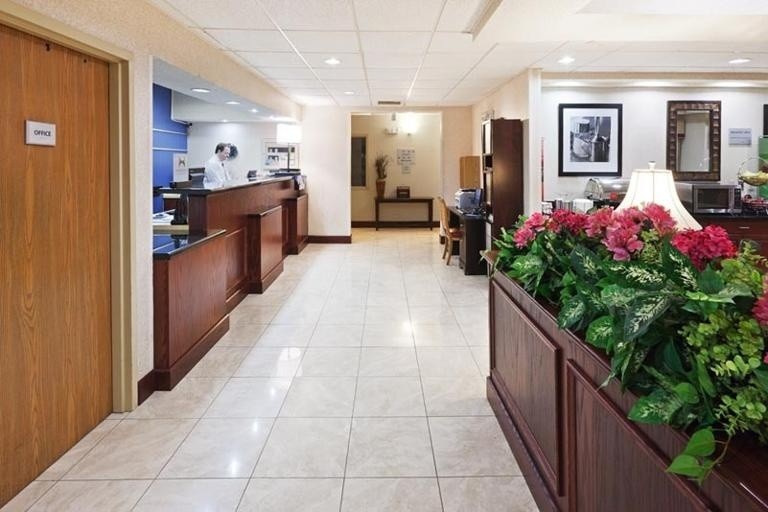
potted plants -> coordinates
[374,152,392,199]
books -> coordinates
[555,197,594,215]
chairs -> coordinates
[438,197,461,266]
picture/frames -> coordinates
[558,104,622,176]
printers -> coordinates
[584,178,630,203]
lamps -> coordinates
[275,124,301,176]
[611,161,703,232]
[399,112,419,136]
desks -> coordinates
[376,197,434,231]
[448,205,486,276]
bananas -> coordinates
[742,169,768,177]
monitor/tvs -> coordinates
[247,169,258,178]
[188,167,204,189]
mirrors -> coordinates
[666,100,721,182]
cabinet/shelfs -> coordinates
[696,214,768,270]
[481,118,523,276]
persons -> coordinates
[203,142,231,189]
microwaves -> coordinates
[672,180,743,216]
